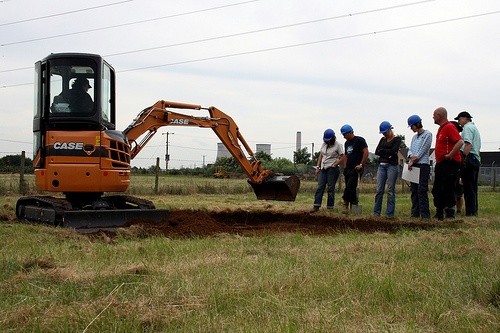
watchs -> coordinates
[359,163,364,167]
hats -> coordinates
[454,111,473,120]
[71,77,92,88]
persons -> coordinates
[432,108,464,221]
[340,124,369,214]
[448,121,464,214]
[55,76,95,113]
[405,115,433,219]
[375,120,401,220]
[310,129,344,214]
[449,112,481,216]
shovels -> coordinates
[352,173,362,217]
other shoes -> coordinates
[432,209,444,221]
[445,209,455,218]
[310,207,319,213]
[349,204,353,213]
[341,202,349,214]
[327,208,333,210]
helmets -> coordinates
[340,124,353,134]
[379,121,392,134]
[408,115,422,128]
[323,129,335,141]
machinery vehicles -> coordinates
[15,52,301,229]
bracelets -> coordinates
[317,166,319,167]
